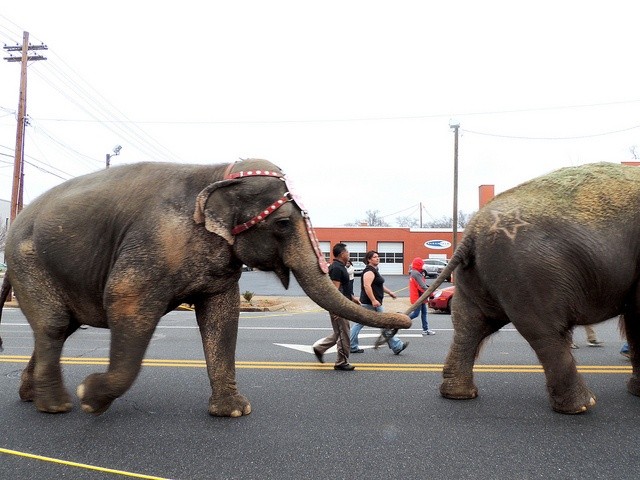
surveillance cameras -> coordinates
[112,144,122,154]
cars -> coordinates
[408,258,448,277]
[429,286,455,314]
[351,262,366,274]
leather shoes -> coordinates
[349,348,364,354]
[312,346,325,364]
[394,340,409,355]
[334,364,355,371]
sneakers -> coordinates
[423,330,436,336]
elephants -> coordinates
[372,162,640,414]
[0,158,412,417]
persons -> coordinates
[313,244,362,371]
[344,261,355,296]
[568,324,603,348]
[408,257,436,335]
[349,251,409,355]
